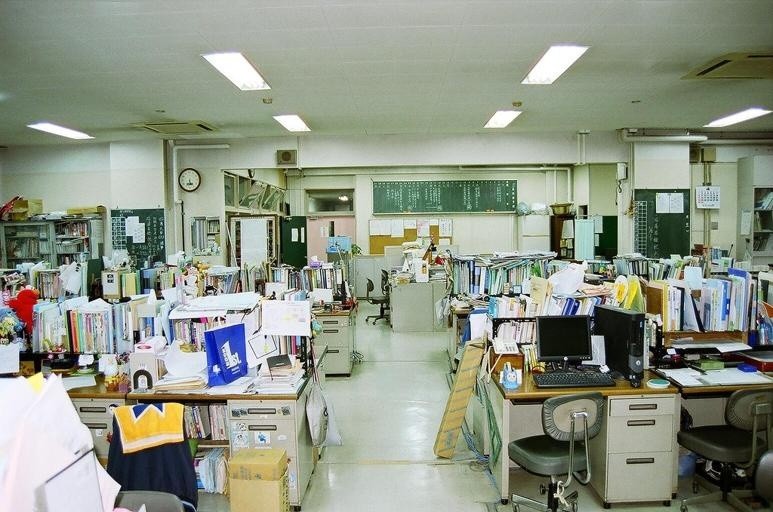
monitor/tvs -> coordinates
[536,315,593,373]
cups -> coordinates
[503,367,517,389]
[104,361,129,391]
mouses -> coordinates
[630,379,641,387]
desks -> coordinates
[654,365,773,398]
[313,345,330,368]
[123,376,318,512]
[447,306,469,374]
[487,371,679,510]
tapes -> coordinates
[505,361,511,372]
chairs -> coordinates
[506,389,604,511]
[113,489,192,512]
[379,268,388,294]
[673,385,772,512]
[752,447,773,512]
[361,276,391,325]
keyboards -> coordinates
[533,373,615,388]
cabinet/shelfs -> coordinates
[547,215,576,260]
[0,218,56,271]
[389,279,449,334]
[49,213,103,262]
[736,152,773,266]
[66,373,129,462]
[311,308,356,377]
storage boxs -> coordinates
[222,467,292,512]
[223,447,289,480]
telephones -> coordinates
[491,336,520,355]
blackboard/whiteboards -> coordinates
[632,186,693,258]
[372,179,517,215]
[110,209,166,268]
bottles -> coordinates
[431,246,438,260]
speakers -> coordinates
[277,150,297,164]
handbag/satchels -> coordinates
[203,315,250,387]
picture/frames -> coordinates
[5,354,42,380]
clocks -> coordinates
[176,166,202,193]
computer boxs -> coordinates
[594,305,645,379]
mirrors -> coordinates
[189,215,221,257]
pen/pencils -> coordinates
[728,244,733,256]
[114,371,127,379]
